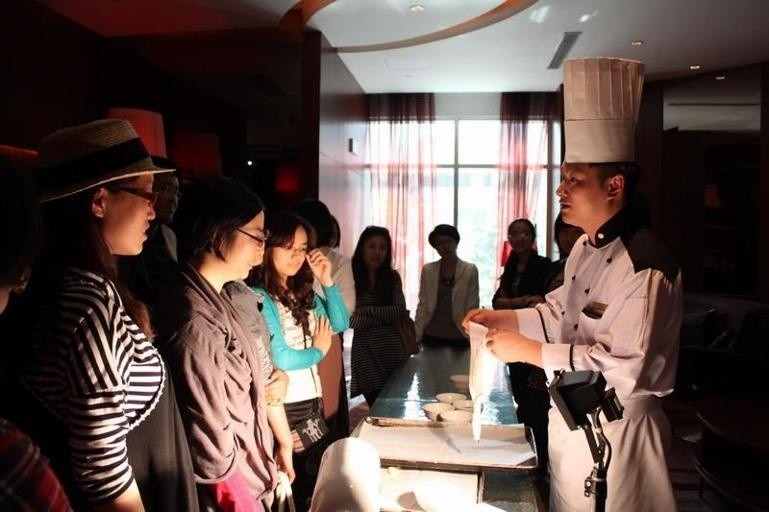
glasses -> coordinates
[239,229,270,247]
[113,187,161,208]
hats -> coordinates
[35,117,177,202]
[562,57,646,165]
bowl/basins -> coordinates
[422,392,475,423]
[450,374,469,390]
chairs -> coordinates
[657,297,767,511]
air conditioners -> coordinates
[657,76,765,137]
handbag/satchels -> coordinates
[293,400,330,454]
[397,310,419,355]
